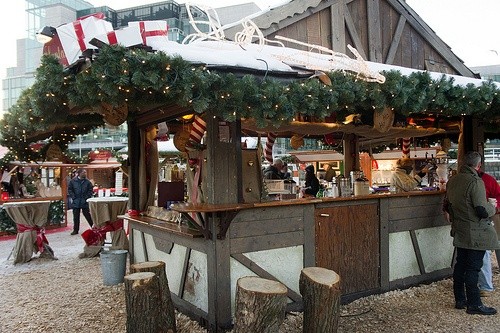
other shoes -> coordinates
[71,231,78,235]
[456,302,469,309]
[480,289,496,297]
[466,306,498,316]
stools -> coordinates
[299,267,343,332]
[233,276,288,333]
[126,260,177,333]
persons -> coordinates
[389,158,426,192]
[323,163,336,182]
[301,165,320,198]
[263,158,290,180]
[441,151,500,315]
[67,169,93,235]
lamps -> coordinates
[36,24,56,44]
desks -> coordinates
[4,202,58,264]
[83,197,131,258]
[116,214,203,238]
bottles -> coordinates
[331,169,370,198]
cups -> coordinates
[488,198,496,206]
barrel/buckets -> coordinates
[99,249,128,285]
